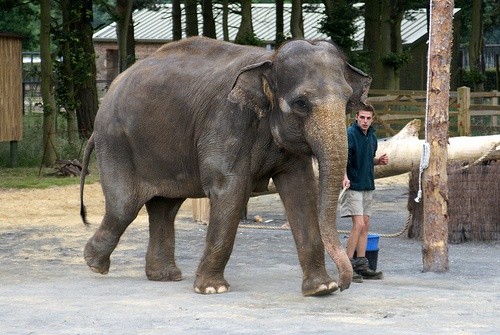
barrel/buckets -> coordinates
[353,235,381,272]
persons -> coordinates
[340,103,389,283]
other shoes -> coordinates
[348,256,382,283]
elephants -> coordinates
[79,34,373,297]
